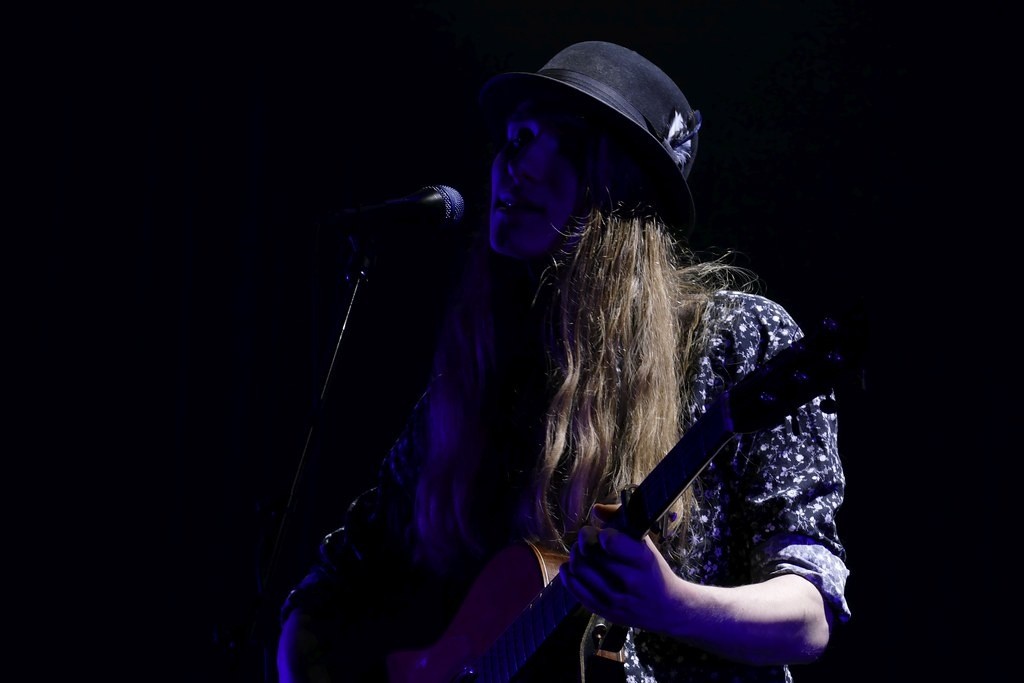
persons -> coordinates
[276,42,857,679]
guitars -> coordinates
[385,312,862,682]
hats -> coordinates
[486,40,702,242]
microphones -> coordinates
[345,184,465,252]
